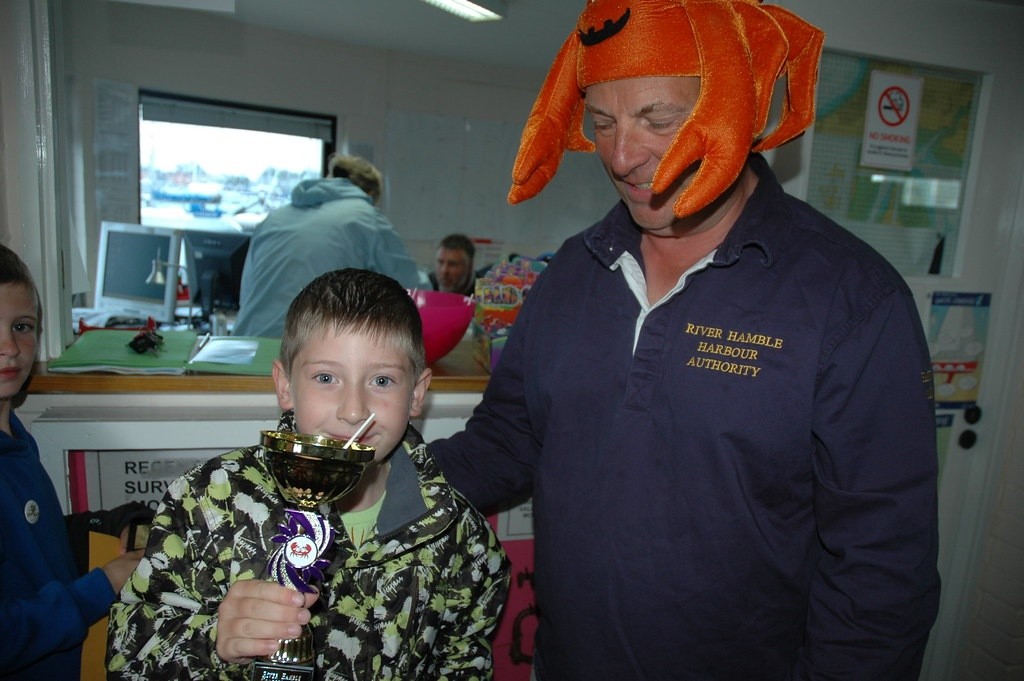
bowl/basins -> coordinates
[406,289,476,366]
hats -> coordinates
[508,0,828,219]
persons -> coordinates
[0,244,145,681]
[427,234,480,297]
[232,155,432,338]
[105,268,511,680]
[428,0,942,680]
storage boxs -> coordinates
[472,319,507,374]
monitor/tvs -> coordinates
[94,222,252,335]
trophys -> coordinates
[252,430,375,681]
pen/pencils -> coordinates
[195,331,212,353]
